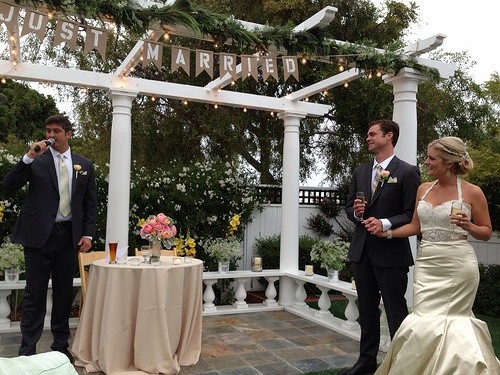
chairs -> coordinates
[78,251,107,319]
[135,248,177,257]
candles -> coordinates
[352,277,356,289]
[254,258,261,270]
[305,265,314,276]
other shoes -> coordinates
[64,350,73,363]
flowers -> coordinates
[0,243,26,273]
[310,237,351,271]
[139,213,177,241]
[73,163,84,178]
[203,234,240,261]
[379,169,390,187]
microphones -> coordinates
[34,138,55,152]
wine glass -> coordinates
[356,192,366,222]
[447,201,462,239]
[141,246,152,263]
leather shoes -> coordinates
[348,362,377,375]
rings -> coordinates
[461,221,462,225]
[372,226,376,229]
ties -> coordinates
[59,154,72,218]
[371,165,380,199]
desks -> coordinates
[71,255,204,375]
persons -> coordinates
[340,121,421,375]
[374,137,500,375]
[0,114,97,360]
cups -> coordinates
[108,239,119,264]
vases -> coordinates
[218,261,229,274]
[5,270,19,284]
[327,269,339,283]
[152,240,161,265]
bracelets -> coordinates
[386,229,393,239]
